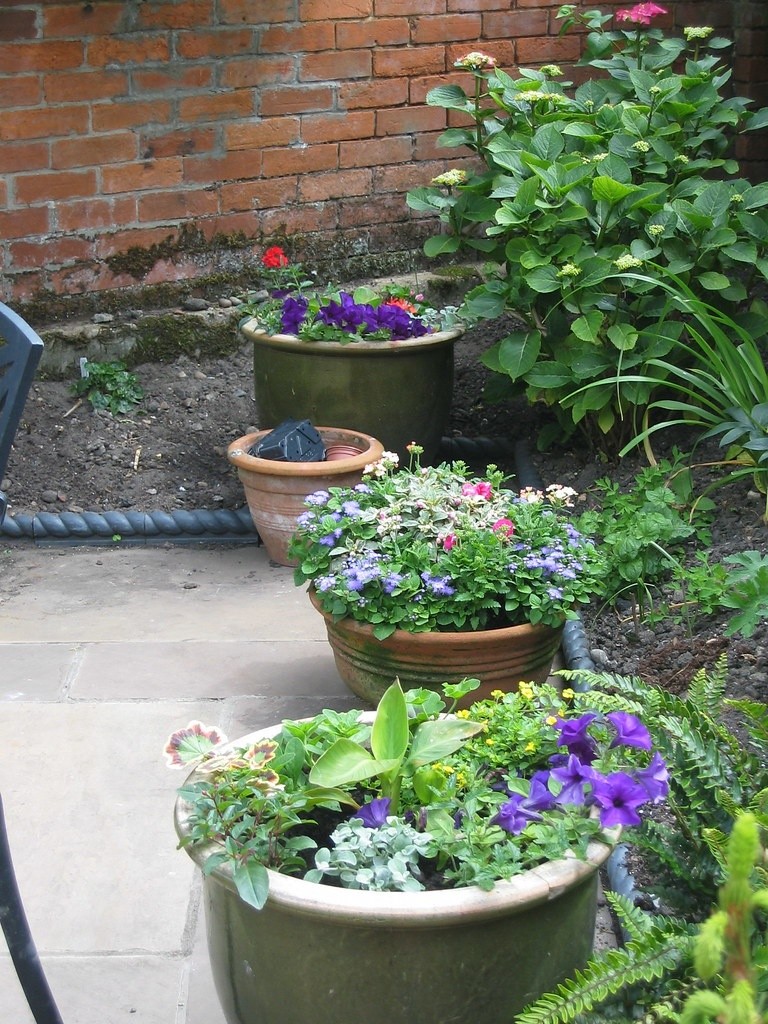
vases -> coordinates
[243,310,469,474]
[228,427,384,567]
[174,711,624,1024]
[307,578,577,713]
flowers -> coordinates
[245,243,440,342]
[282,441,611,642]
[161,672,675,911]
